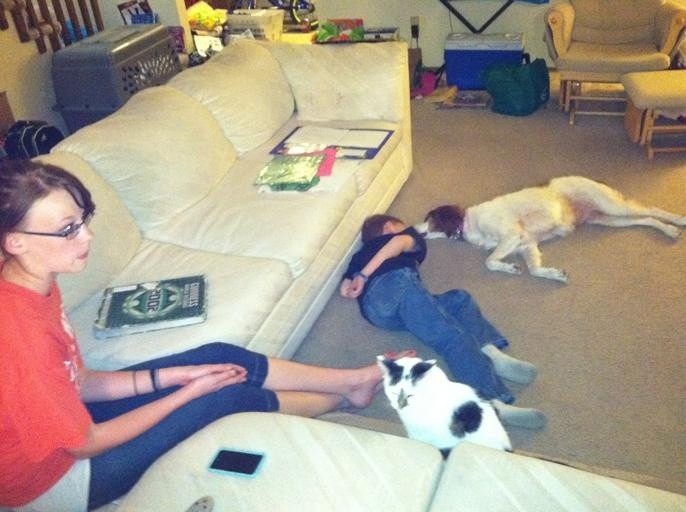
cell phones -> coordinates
[208,445,268,480]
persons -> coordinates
[0,160,416,511]
[339,214,549,430]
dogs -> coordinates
[413,175,686,283]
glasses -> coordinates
[20,211,93,241]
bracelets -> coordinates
[150,367,162,393]
[352,273,369,282]
[133,369,140,397]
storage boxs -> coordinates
[443,32,526,92]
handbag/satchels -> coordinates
[483,53,550,116]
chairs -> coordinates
[541,1,686,126]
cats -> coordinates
[376,354,514,458]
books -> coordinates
[425,85,492,107]
[92,274,209,340]
[253,124,393,192]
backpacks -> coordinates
[4,118,64,160]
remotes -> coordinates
[185,494,216,512]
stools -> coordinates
[622,69,686,161]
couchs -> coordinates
[0,37,686,511]
